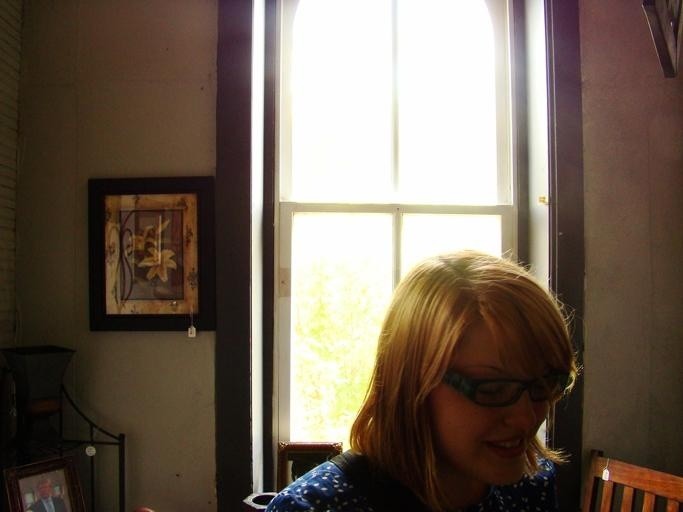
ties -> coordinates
[46,500,54,512]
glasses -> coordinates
[442,368,569,407]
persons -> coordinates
[29,478,67,512]
[265,248,583,512]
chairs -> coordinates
[581,448,682,512]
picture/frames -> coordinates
[86,175,216,333]
[1,453,88,512]
[276,443,339,494]
